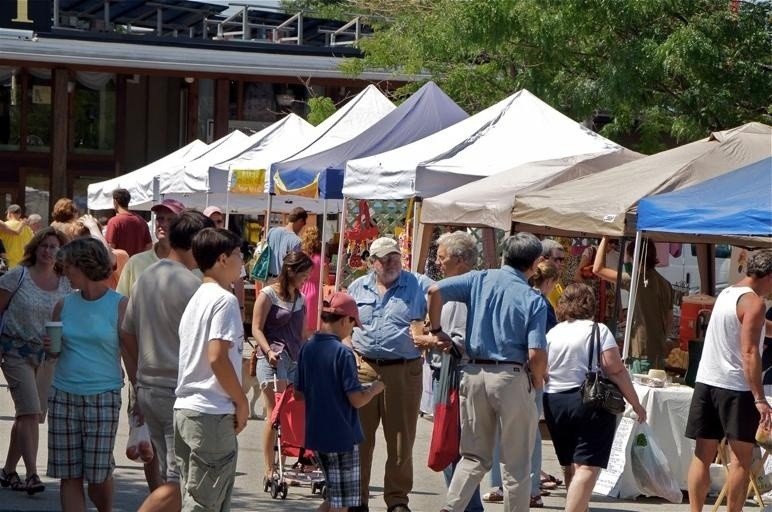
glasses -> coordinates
[545,255,565,264]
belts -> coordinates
[354,350,420,367]
[468,358,525,367]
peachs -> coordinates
[126,441,153,462]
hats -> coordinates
[369,236,402,259]
[151,198,185,215]
[322,292,365,332]
[202,205,223,217]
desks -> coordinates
[622,374,695,497]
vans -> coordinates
[575,238,732,337]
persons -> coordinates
[2,227,76,494]
[251,207,429,511]
[592,234,675,375]
[115,201,249,511]
[76,214,130,292]
[0,189,152,257]
[415,230,647,510]
[43,238,145,511]
[683,248,771,511]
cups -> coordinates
[410,318,423,348]
[44,320,63,353]
[754,421,771,443]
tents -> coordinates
[87,80,771,366]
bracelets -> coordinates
[266,349,272,357]
[430,326,442,334]
[240,306,245,309]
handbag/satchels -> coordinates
[344,226,378,270]
[250,351,256,377]
[427,390,459,472]
[580,268,598,280]
[248,245,272,283]
[580,371,626,415]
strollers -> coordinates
[263,354,328,500]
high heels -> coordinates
[1,468,27,492]
[25,473,45,496]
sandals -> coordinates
[482,473,562,507]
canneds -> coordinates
[754,420,771,444]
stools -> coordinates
[711,436,772,512]
